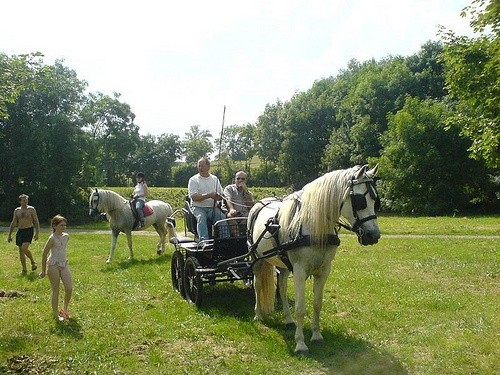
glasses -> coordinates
[236,178,246,181]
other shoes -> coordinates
[31,261,37,270]
[22,269,27,275]
[59,308,68,321]
[140,221,144,227]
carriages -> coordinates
[163,162,381,356]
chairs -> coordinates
[184,201,197,234]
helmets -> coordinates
[135,172,145,178]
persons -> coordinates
[130,172,151,227]
[7,194,40,274]
[39,215,73,323]
[188,157,230,250]
[220,171,256,237]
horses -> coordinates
[89,187,175,264]
[247,162,380,353]
